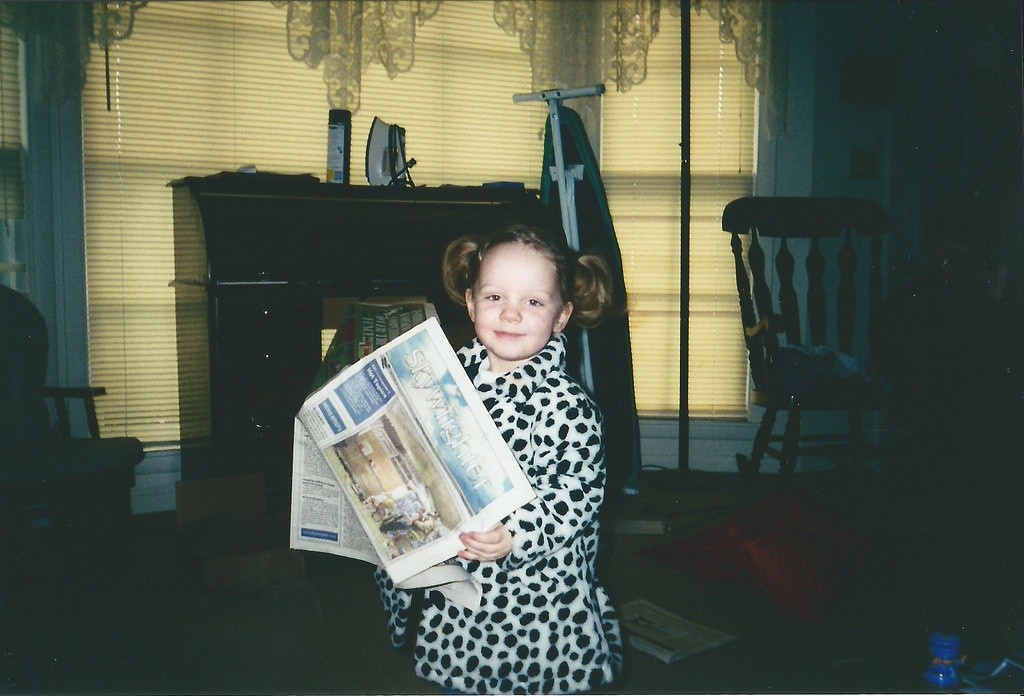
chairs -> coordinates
[724,197,907,477]
[0,286,145,528]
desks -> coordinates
[171,170,542,448]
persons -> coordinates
[379,508,435,555]
[373,225,623,695]
